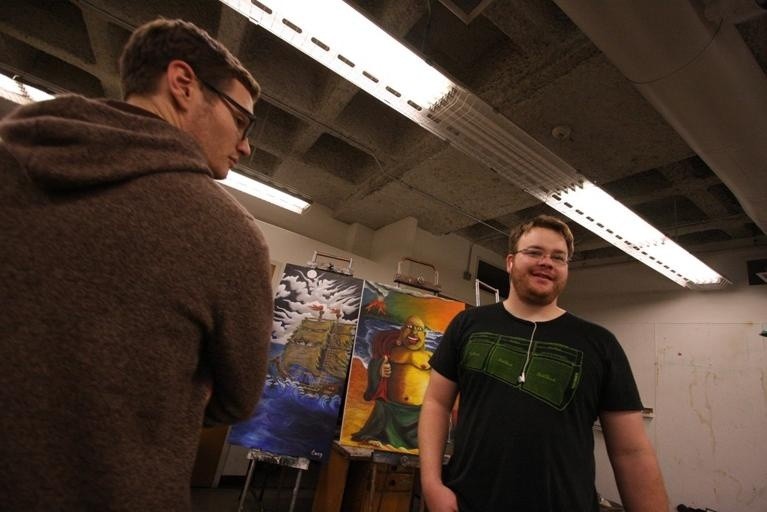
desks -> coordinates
[311,441,448,512]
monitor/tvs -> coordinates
[473,256,510,299]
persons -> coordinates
[350,313,434,449]
[417,215,670,512]
[0,18,273,511]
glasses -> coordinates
[201,79,258,140]
[513,247,570,265]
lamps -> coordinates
[218,0,734,291]
[0,74,312,215]
